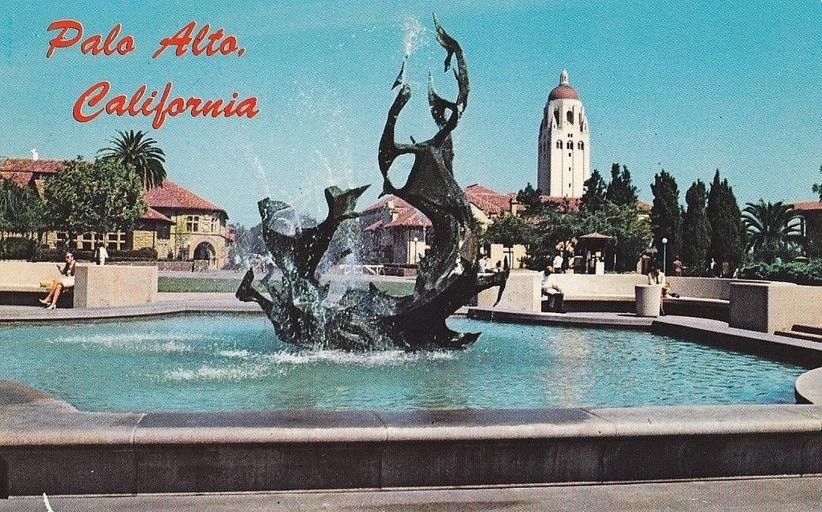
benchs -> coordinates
[0,284,72,293]
[542,295,730,309]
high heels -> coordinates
[38,299,56,310]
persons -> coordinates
[37,252,81,311]
[538,263,567,314]
[647,265,668,315]
[479,250,718,277]
[94,242,110,265]
[233,253,242,273]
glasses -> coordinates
[64,256,71,259]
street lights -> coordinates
[413,237,418,264]
[662,237,668,275]
